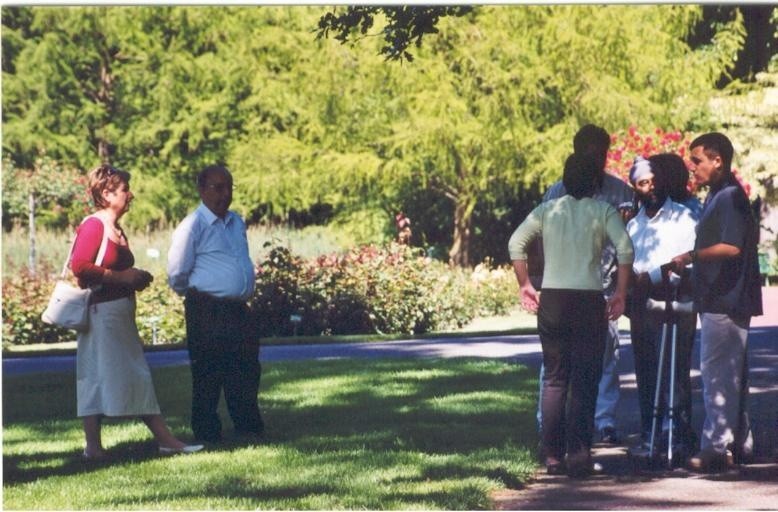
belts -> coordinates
[188,287,247,305]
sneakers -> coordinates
[538,425,755,479]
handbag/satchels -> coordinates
[41,213,107,331]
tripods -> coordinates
[647,315,678,471]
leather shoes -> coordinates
[159,443,204,456]
[83,450,113,459]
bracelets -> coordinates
[688,249,698,265]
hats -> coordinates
[630,156,659,185]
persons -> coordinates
[71,164,205,460]
[671,132,763,471]
[507,153,635,475]
[165,165,265,442]
[626,152,704,463]
[526,124,637,442]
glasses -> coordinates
[107,167,118,175]
[206,184,233,191]
